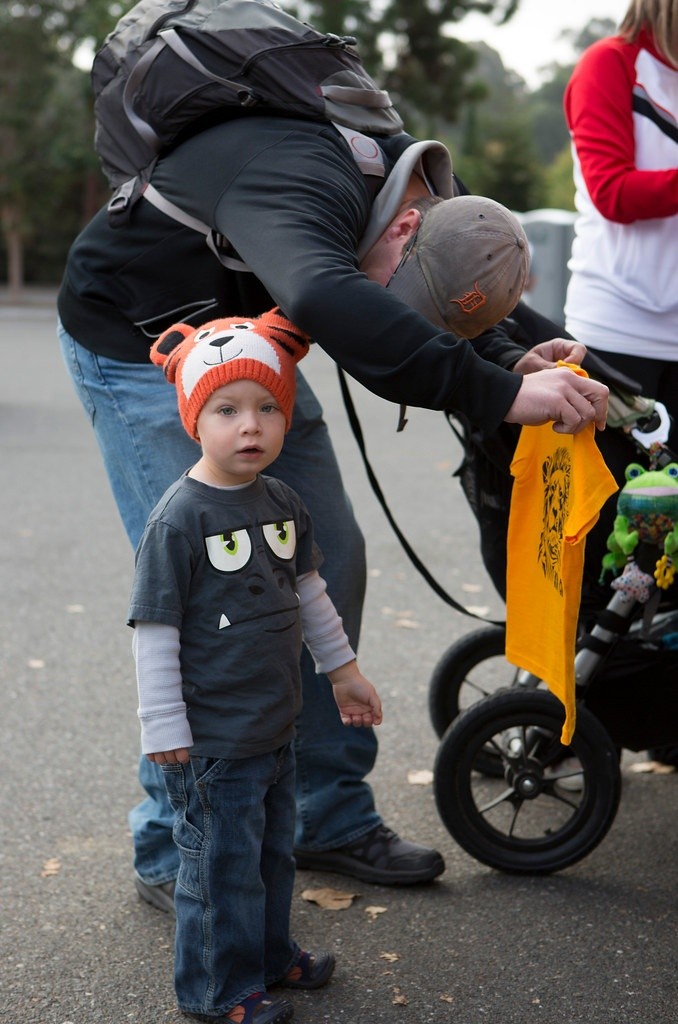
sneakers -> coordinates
[136,871,183,918]
[280,949,334,989]
[184,990,292,1024]
[295,818,445,885]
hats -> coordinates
[384,196,533,341]
[148,306,307,438]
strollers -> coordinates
[424,373,678,881]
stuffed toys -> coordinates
[599,462,678,603]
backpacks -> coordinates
[92,0,415,253]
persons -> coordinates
[564,0,678,458]
[126,304,382,1024]
[56,121,611,920]
